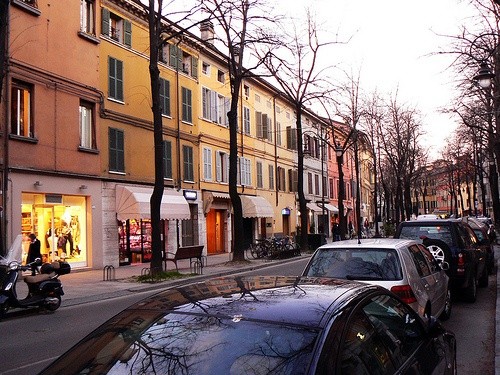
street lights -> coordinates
[335,141,345,241]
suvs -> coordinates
[392,220,490,301]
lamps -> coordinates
[33,180,43,187]
[79,184,88,189]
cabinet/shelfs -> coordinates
[21,217,38,233]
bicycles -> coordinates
[247,234,302,260]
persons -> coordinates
[26,234,41,276]
[480,218,496,241]
[348,221,352,234]
[309,223,315,233]
[318,224,324,233]
[364,218,368,228]
[45,231,58,250]
[332,223,338,242]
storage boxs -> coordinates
[53,261,71,275]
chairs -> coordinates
[348,257,364,264]
[229,353,288,375]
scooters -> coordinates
[0,256,71,315]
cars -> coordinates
[39,275,458,375]
[298,232,454,324]
[416,214,443,233]
[462,216,494,271]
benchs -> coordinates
[166,245,207,269]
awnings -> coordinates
[307,203,322,211]
[324,204,338,211]
[203,190,232,214]
[239,194,274,218]
[115,184,190,220]
